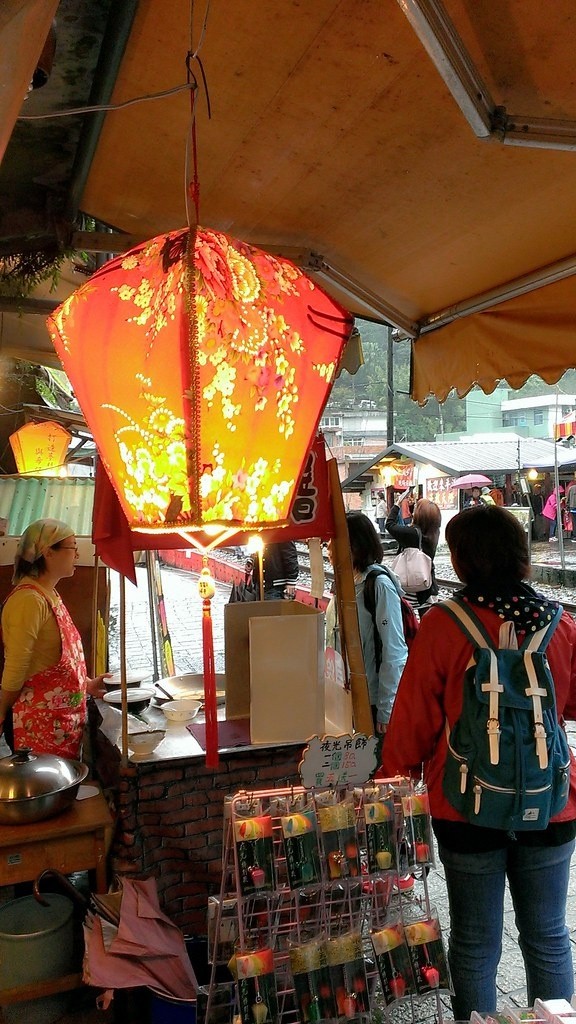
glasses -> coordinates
[54,545,79,554]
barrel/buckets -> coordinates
[0,893,79,1024]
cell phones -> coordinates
[409,485,415,493]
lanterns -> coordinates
[46,227,354,771]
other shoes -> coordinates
[570,540,576,543]
[549,537,558,542]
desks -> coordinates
[0,780,113,1024]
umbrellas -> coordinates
[450,473,493,502]
[229,561,256,603]
[32,869,197,1011]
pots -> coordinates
[152,674,226,706]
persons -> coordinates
[374,493,388,539]
[463,486,487,509]
[533,484,546,540]
[244,541,299,601]
[372,505,576,1024]
[395,492,415,525]
[385,498,441,619]
[542,486,566,542]
[565,485,576,542]
[481,487,496,505]
[324,512,409,774]
[0,519,114,758]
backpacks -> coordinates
[364,565,419,661]
[432,596,572,830]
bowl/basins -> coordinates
[0,759,89,825]
[109,698,151,715]
[161,701,202,721]
[104,681,140,693]
[127,733,165,755]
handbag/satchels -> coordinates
[563,513,573,531]
[391,528,432,592]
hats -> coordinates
[481,487,491,495]
